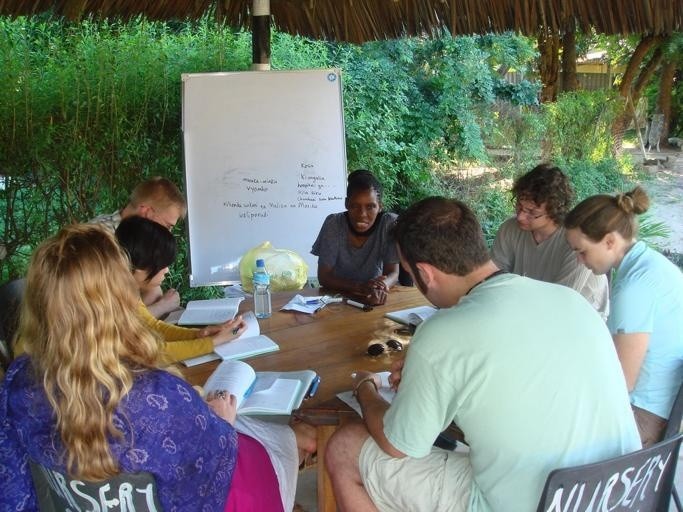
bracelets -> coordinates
[352,377,379,399]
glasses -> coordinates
[367,340,403,356]
[511,194,548,220]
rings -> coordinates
[216,391,226,399]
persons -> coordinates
[0,222,317,512]
[323,195,646,511]
[115,215,178,323]
[87,177,189,320]
[309,169,403,307]
[562,186,683,451]
[490,162,609,324]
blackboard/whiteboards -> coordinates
[180,69,348,288]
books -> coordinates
[176,296,244,327]
[208,310,280,362]
[384,304,440,326]
[253,368,316,410]
[203,358,301,417]
[180,350,219,368]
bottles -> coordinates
[252,259,272,319]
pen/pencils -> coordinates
[175,282,181,292]
[299,299,322,304]
[233,324,241,335]
[309,377,320,397]
[345,298,373,312]
[244,376,259,398]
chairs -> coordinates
[25,454,165,511]
[1,276,29,378]
[536,378,683,511]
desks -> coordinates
[170,284,439,512]
